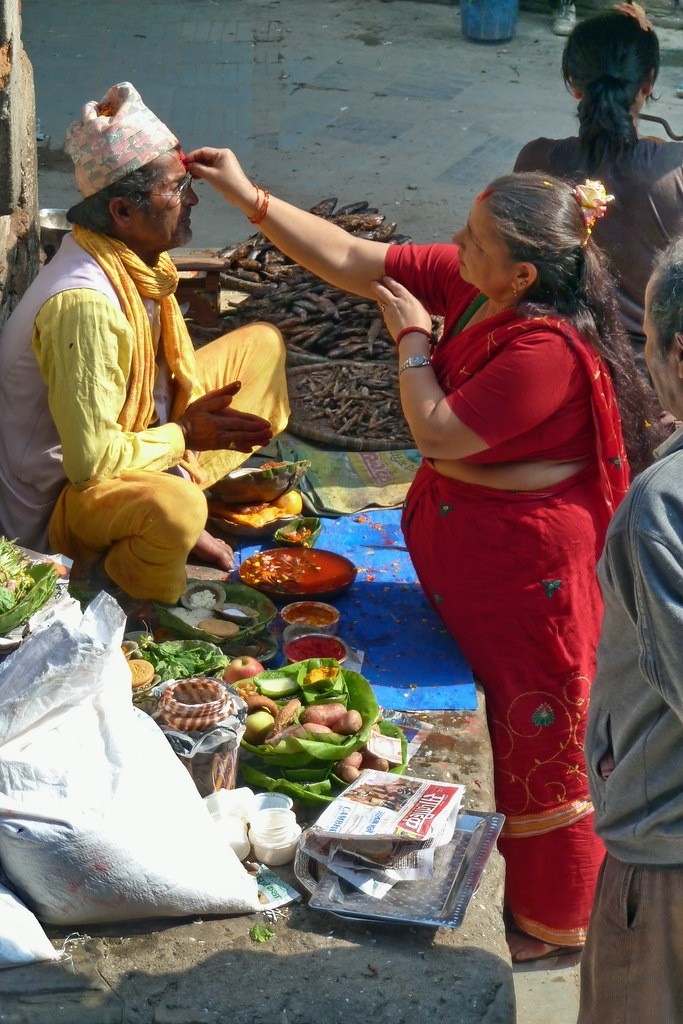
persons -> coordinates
[0,80,291,623]
[512,10,683,370]
[184,144,634,962]
[576,237,683,1024]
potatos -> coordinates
[265,698,363,748]
[334,751,390,786]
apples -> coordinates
[223,656,265,683]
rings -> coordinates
[380,304,385,311]
[228,440,236,450]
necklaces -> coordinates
[455,290,483,316]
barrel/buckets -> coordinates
[460,0,521,45]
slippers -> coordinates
[502,916,583,964]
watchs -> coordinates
[399,354,433,375]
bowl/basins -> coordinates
[274,515,323,550]
[284,630,350,670]
[279,600,341,647]
[203,780,303,870]
[38,204,81,256]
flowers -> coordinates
[576,177,615,228]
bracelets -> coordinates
[396,326,431,349]
[247,183,270,224]
[181,424,191,450]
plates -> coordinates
[205,455,310,537]
[236,542,358,603]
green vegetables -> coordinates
[138,633,229,682]
[0,535,36,616]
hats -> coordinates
[66,79,180,200]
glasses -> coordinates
[137,170,192,201]
